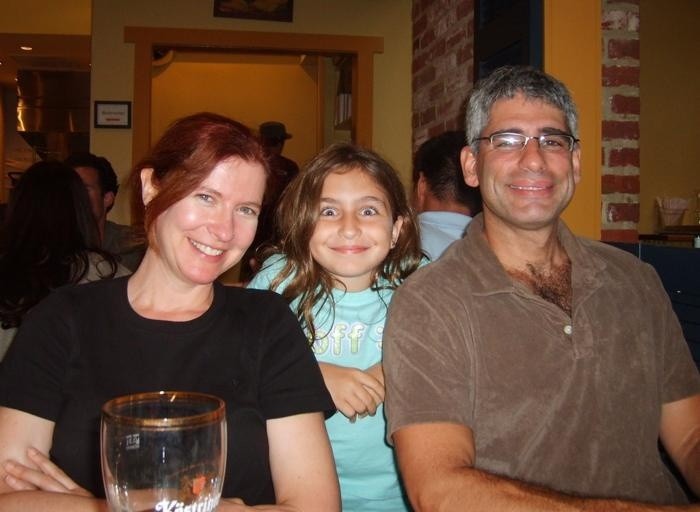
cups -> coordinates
[653,208,687,235]
[101,392,228,511]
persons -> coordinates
[69,151,148,274]
[238,120,300,284]
[380,62,699,512]
[243,138,434,511]
[0,158,134,361]
[1,110,344,512]
[407,128,483,264]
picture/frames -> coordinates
[213,1,294,24]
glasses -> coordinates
[472,132,580,153]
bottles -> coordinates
[693,233,700,248]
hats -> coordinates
[259,121,294,143]
